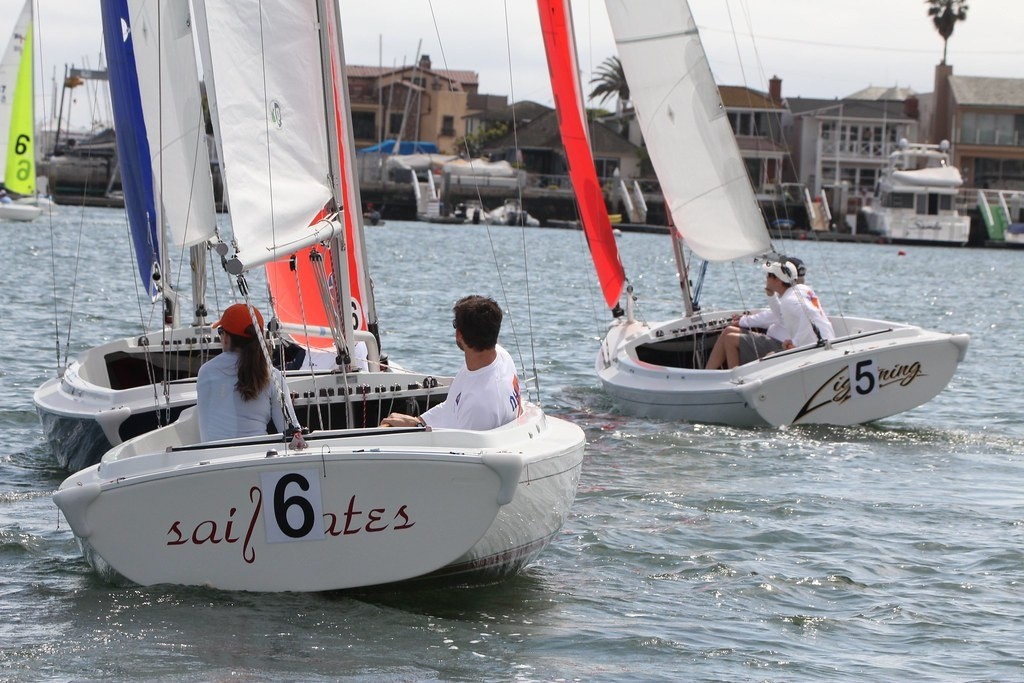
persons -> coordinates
[381,295,521,431]
[366,202,379,225]
[196,304,301,444]
[0,189,12,203]
[705,257,835,370]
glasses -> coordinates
[453,319,460,329]
[217,327,227,335]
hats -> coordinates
[786,257,806,276]
[212,304,264,338]
[763,261,798,284]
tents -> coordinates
[359,140,438,154]
[389,154,513,176]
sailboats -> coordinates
[0,0,43,221]
[593,0,969,435]
[36,0,585,595]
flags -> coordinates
[66,78,83,87]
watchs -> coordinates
[416,422,423,427]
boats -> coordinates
[491,199,540,226]
[363,217,384,226]
[453,201,493,226]
[861,138,971,248]
[16,196,58,215]
[608,214,623,223]
[769,217,794,229]
